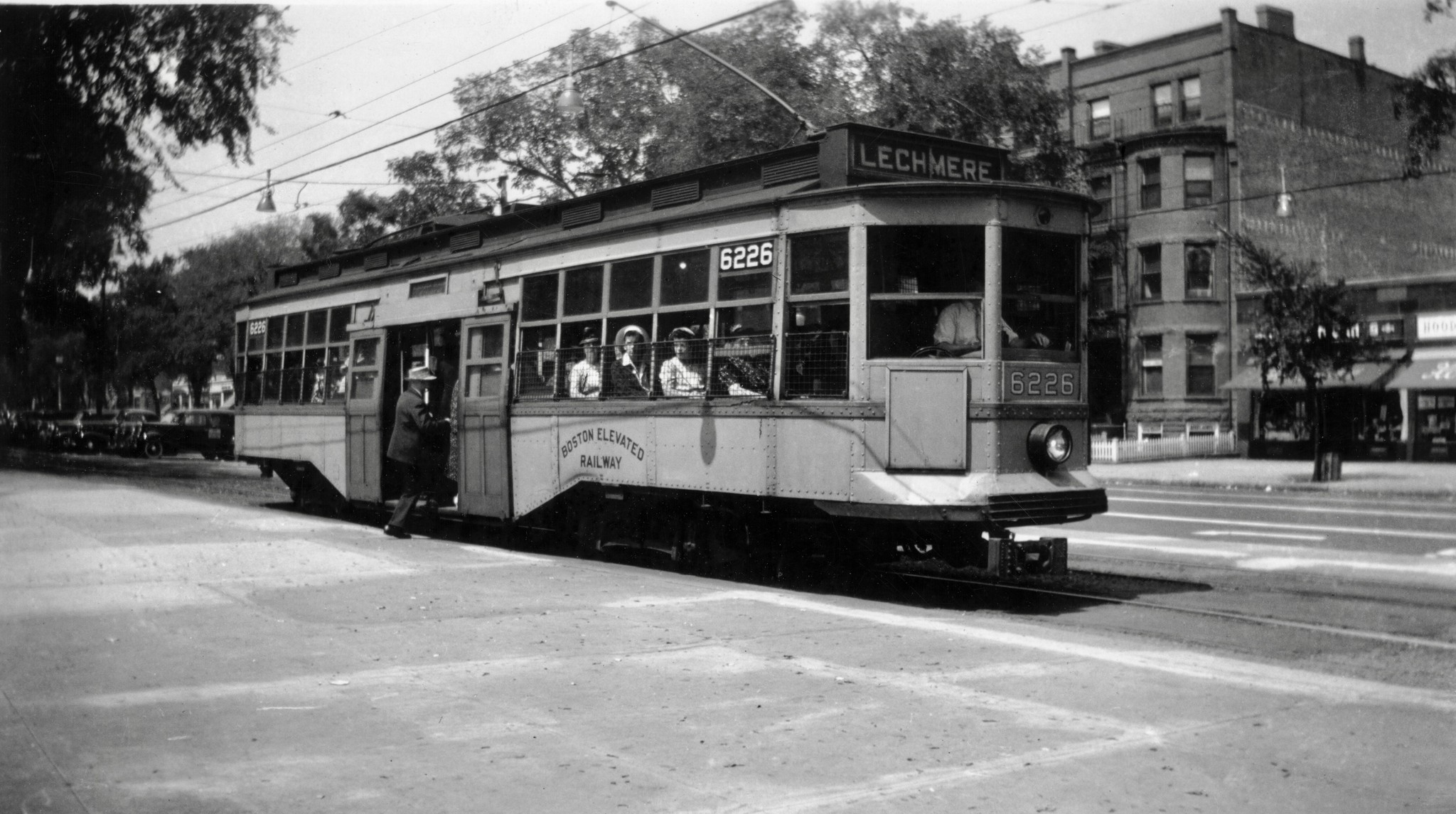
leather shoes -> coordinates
[383,525,412,539]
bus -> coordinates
[227,0,1112,608]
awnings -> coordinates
[1219,359,1396,390]
[1384,359,1456,388]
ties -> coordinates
[975,307,982,342]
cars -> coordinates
[0,404,235,462]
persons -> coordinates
[658,327,706,399]
[383,352,452,539]
[929,274,1050,358]
[610,325,653,400]
[568,328,602,400]
[717,323,770,398]
[546,356,577,401]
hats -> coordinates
[405,366,437,382]
[579,327,600,345]
[668,327,695,341]
[728,324,753,342]
[615,325,650,353]
